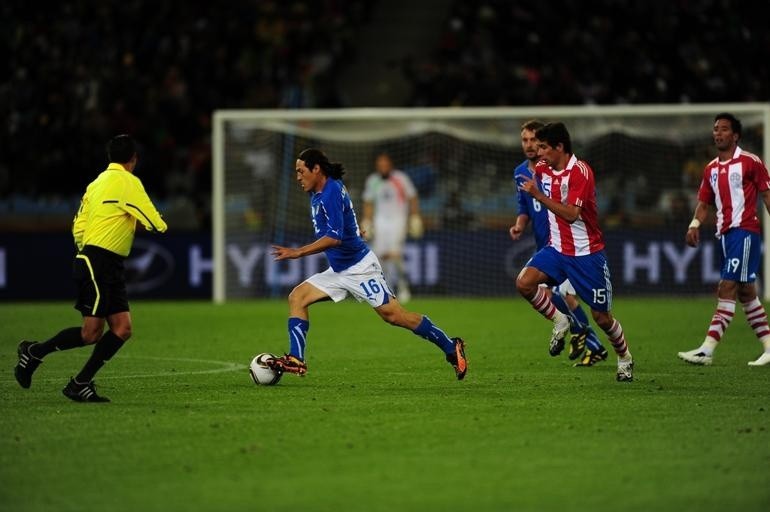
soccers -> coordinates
[249,352,282,384]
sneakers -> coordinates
[572,345,608,367]
[568,321,592,360]
[62,376,111,402]
[14,340,44,389]
[549,314,574,356]
[446,337,467,380]
[747,352,770,366]
[266,352,307,375]
[678,348,714,366]
[617,355,634,382]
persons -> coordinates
[1,1,769,233]
[674,107,770,371]
[506,116,609,369]
[12,132,169,406]
[266,146,468,384]
[360,152,422,307]
[515,121,636,384]
